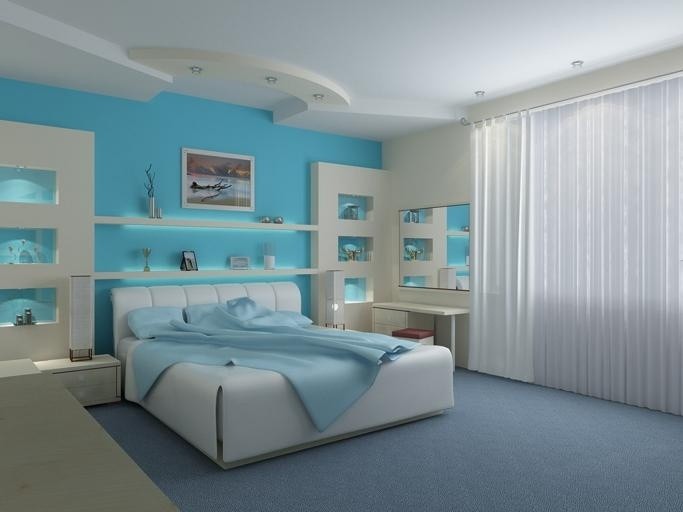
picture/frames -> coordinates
[182,250,198,271]
[180,147,254,212]
[230,257,249,269]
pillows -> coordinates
[126,302,223,340]
[226,295,314,332]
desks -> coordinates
[371,301,470,374]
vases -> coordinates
[148,196,157,218]
[264,256,276,270]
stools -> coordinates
[391,327,434,345]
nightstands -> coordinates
[32,353,121,408]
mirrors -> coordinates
[398,201,470,292]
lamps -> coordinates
[438,268,457,289]
[324,270,345,329]
[68,274,95,362]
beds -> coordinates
[109,280,455,469]
